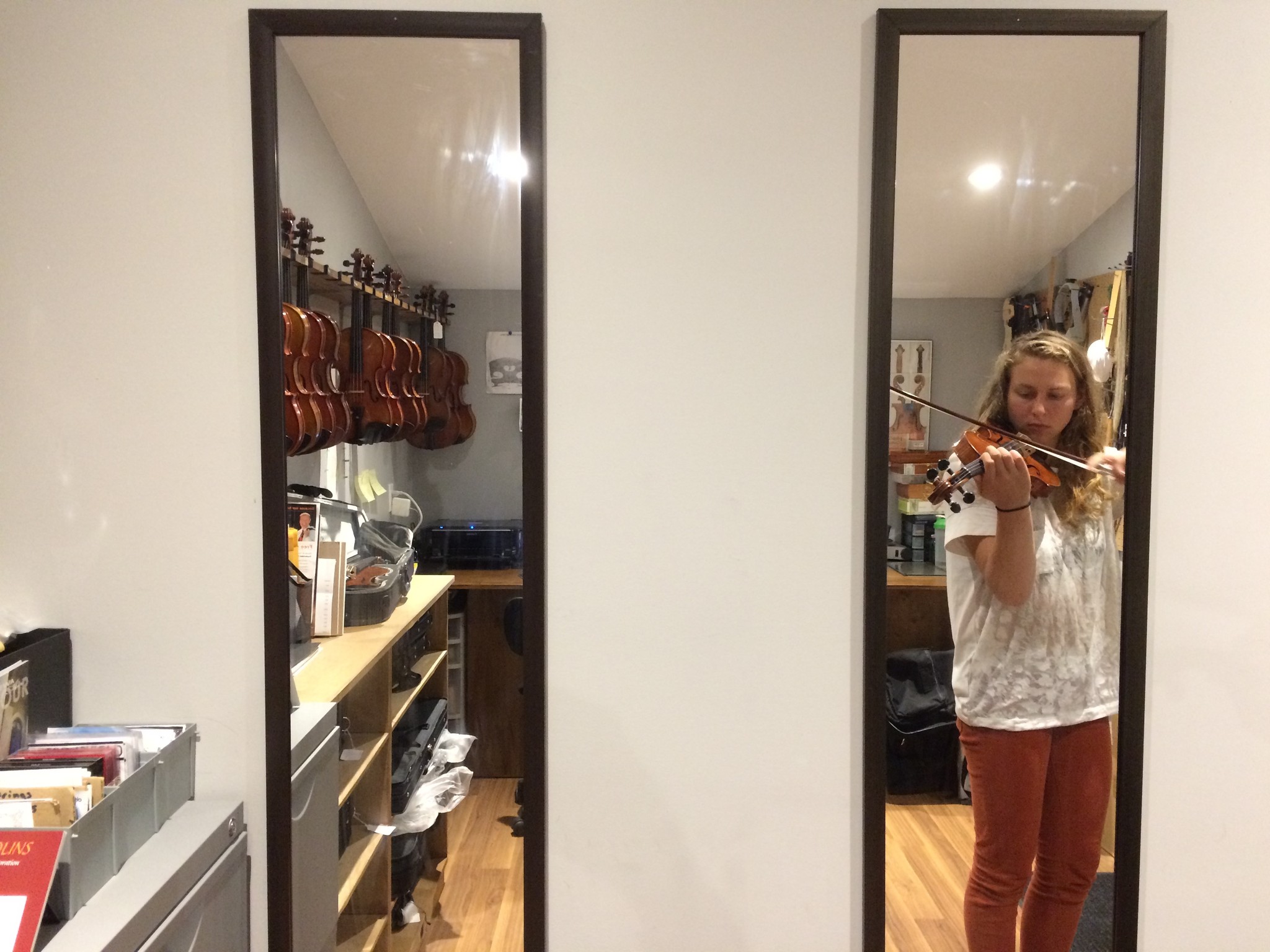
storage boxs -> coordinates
[886,449,950,561]
[0,722,200,923]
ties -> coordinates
[298,530,304,541]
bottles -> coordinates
[934,515,946,569]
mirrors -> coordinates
[858,8,1171,952]
[248,7,552,952]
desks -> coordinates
[882,560,952,657]
[464,566,527,780]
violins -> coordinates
[924,418,1064,514]
[277,201,479,451]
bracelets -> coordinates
[997,500,1032,512]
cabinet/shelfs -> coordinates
[289,702,339,952]
[290,570,455,952]
[33,800,244,952]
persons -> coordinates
[297,513,315,542]
[945,331,1127,952]
